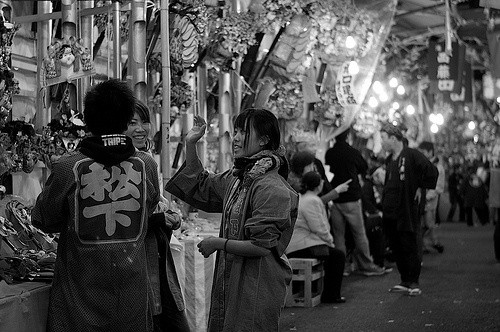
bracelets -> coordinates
[224,239,229,252]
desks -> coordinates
[0,279,52,332]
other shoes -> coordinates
[362,266,385,276]
[407,287,422,296]
[391,283,411,293]
[432,244,444,254]
[343,269,352,277]
[379,265,394,272]
[336,296,346,303]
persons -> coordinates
[162,108,300,332]
[325,128,385,276]
[123,96,192,332]
[283,141,353,304]
[30,78,161,332]
[362,136,491,273]
[379,124,439,297]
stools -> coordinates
[286,258,324,309]
